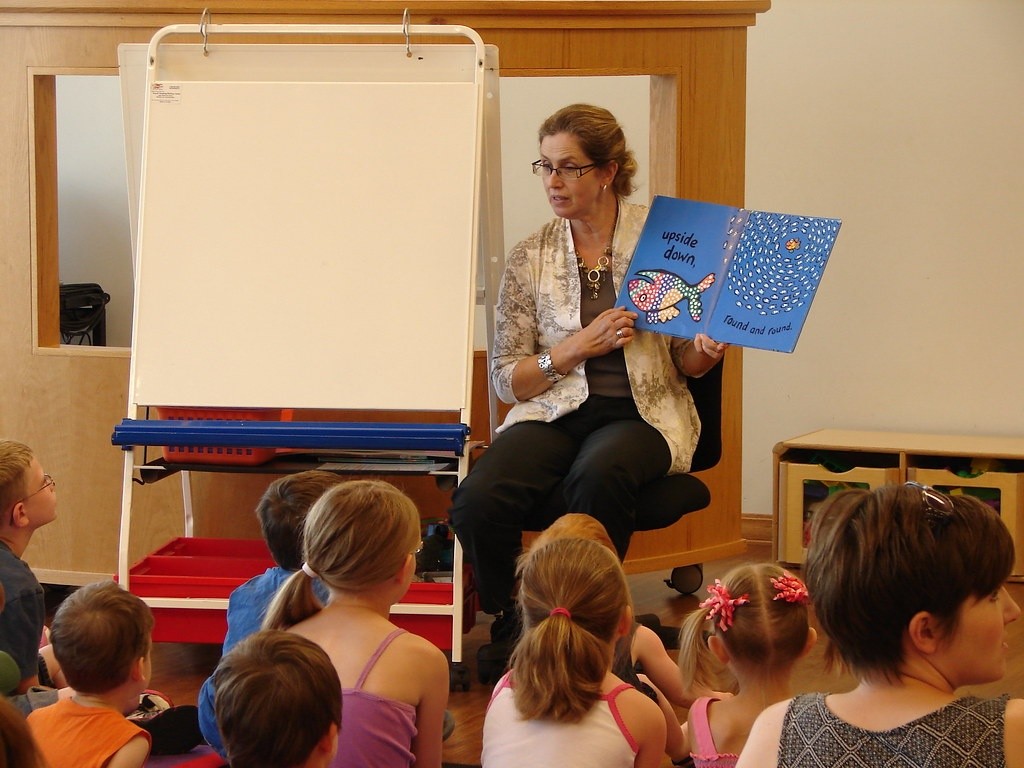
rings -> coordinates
[616,330,623,338]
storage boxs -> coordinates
[394,578,452,648]
[155,409,281,465]
[152,537,270,555]
[110,554,275,644]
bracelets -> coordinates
[537,349,568,382]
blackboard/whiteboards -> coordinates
[118,24,501,409]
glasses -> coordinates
[531,160,598,181]
[9,473,56,526]
[905,480,955,530]
[413,542,423,555]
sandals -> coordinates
[671,754,694,768]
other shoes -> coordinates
[124,689,204,756]
[442,711,455,744]
[490,601,521,642]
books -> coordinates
[614,195,842,353]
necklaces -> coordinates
[575,221,616,300]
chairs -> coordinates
[632,345,723,536]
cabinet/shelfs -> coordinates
[771,428,1024,584]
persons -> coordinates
[213,629,343,768]
[262,481,448,768]
[529,512,733,768]
[198,471,454,758]
[678,563,817,768]
[449,104,730,661]
[733,481,1024,768]
[481,538,667,768]
[0,441,206,768]
